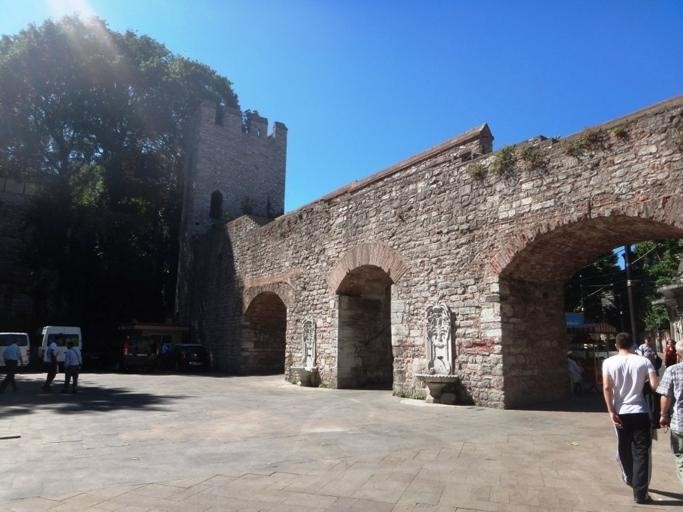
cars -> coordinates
[121,333,216,374]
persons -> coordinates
[637,336,676,374]
[0,338,23,393]
[40,336,60,392]
[602,332,659,504]
[655,340,683,486]
[59,341,82,393]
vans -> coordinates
[0,332,32,373]
[37,326,83,373]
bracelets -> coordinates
[660,412,669,417]
